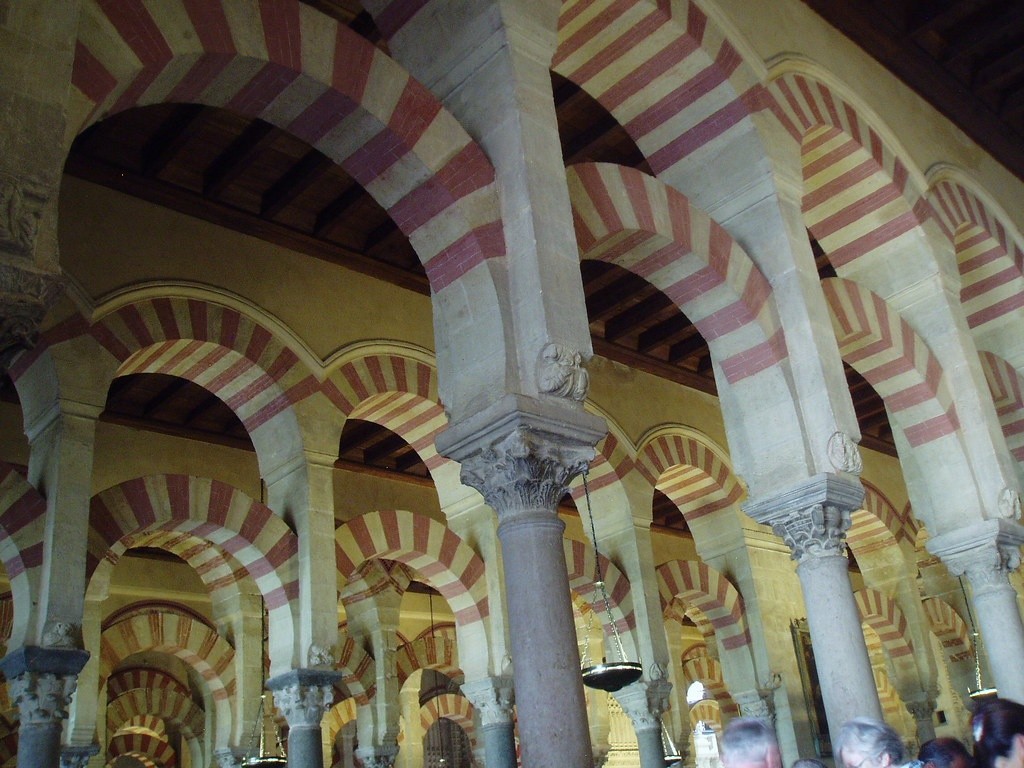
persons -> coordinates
[718,718,782,768]
[917,737,968,768]
[973,699,1024,768]
[836,716,924,768]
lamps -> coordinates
[241,597,288,768]
[580,472,644,693]
[959,575,998,702]
